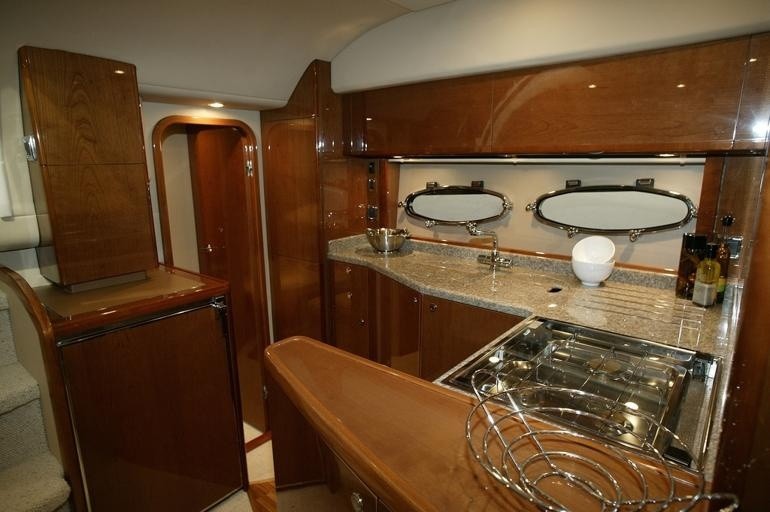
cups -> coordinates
[674,232,708,301]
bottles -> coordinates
[714,215,735,306]
[690,241,722,307]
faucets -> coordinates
[466,222,498,256]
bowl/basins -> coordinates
[571,256,617,288]
[571,235,616,265]
[365,226,410,252]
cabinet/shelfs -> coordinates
[263,366,392,511]
[32,262,249,512]
[330,259,526,384]
